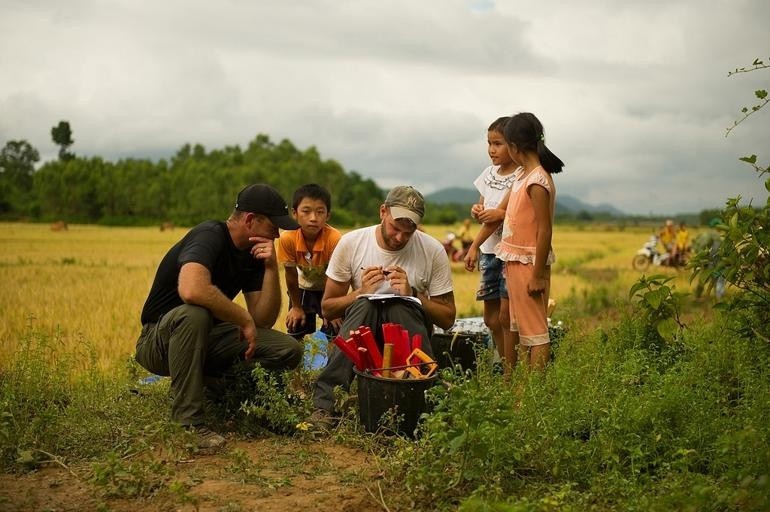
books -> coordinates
[356,292,395,298]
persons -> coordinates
[453,219,471,261]
[657,217,728,308]
[464,112,566,396]
[135,183,303,449]
[277,182,343,347]
[303,184,456,435]
[470,117,523,382]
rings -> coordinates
[260,247,263,252]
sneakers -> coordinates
[185,424,225,449]
[312,408,331,435]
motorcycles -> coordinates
[632,227,690,272]
[442,229,480,263]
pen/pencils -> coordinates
[359,266,392,276]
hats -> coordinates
[384,186,425,224]
[235,184,302,230]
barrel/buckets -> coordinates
[431,331,489,377]
[352,361,444,440]
